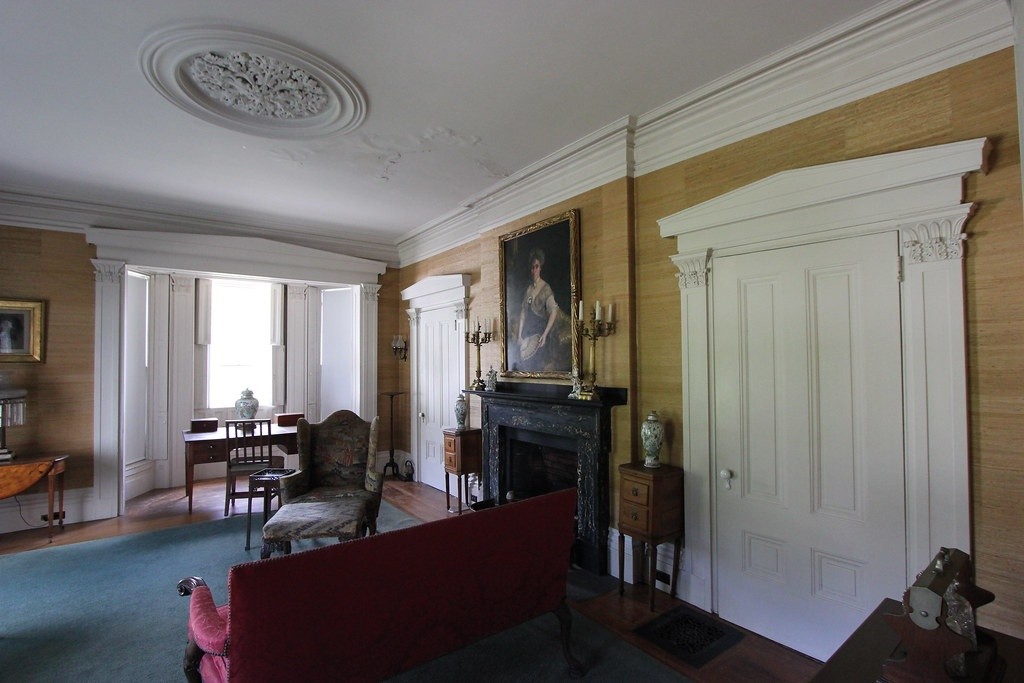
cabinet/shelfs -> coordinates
[618,460,685,613]
[442,426,481,515]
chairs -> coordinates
[223,418,284,516]
[260,409,381,559]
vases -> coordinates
[235,388,259,430]
[641,409,665,469]
[454,397,467,431]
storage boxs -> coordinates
[274,413,305,427]
[191,418,218,433]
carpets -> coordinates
[562,560,627,604]
[630,604,748,671]
[0,498,697,683]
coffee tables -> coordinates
[245,468,296,553]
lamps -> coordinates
[391,335,408,362]
[0,389,28,463]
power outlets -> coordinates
[41,515,49,521]
[53,511,65,520]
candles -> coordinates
[579,300,584,322]
[608,303,612,322]
[595,300,602,324]
[466,316,491,333]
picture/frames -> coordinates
[0,300,45,363]
[499,207,583,380]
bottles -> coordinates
[640,412,664,469]
[234,388,259,425]
[455,395,467,430]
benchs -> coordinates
[174,487,591,683]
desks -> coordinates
[182,423,298,516]
[811,597,1024,683]
[0,452,70,543]
